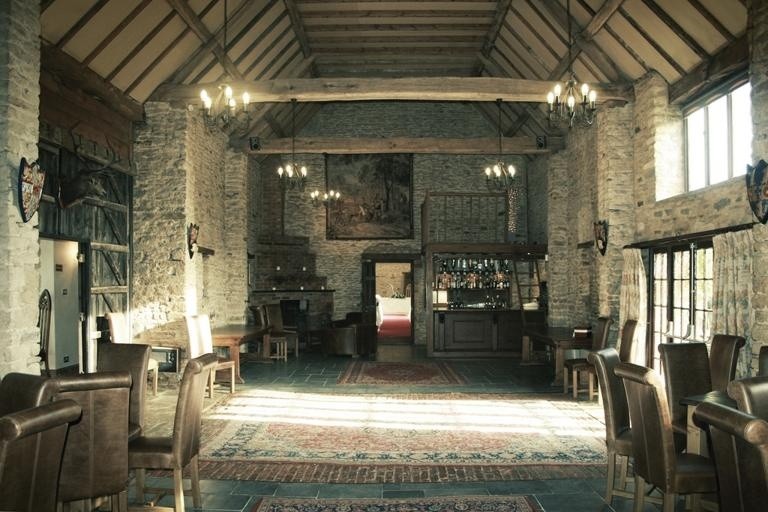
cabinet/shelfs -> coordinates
[432,253,512,309]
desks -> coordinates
[211,323,268,385]
[520,327,592,386]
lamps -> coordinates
[547,0,598,128]
[310,152,342,207]
[484,98,516,193]
[200,0,252,138]
[276,98,308,192]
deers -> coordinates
[57,132,123,209]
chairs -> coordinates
[564,316,768,512]
[1,368,134,512]
[258,304,296,363]
[38,289,52,369]
[129,352,217,512]
[99,342,151,505]
[321,306,377,358]
[183,314,236,398]
[104,312,158,394]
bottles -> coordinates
[432,258,512,309]
